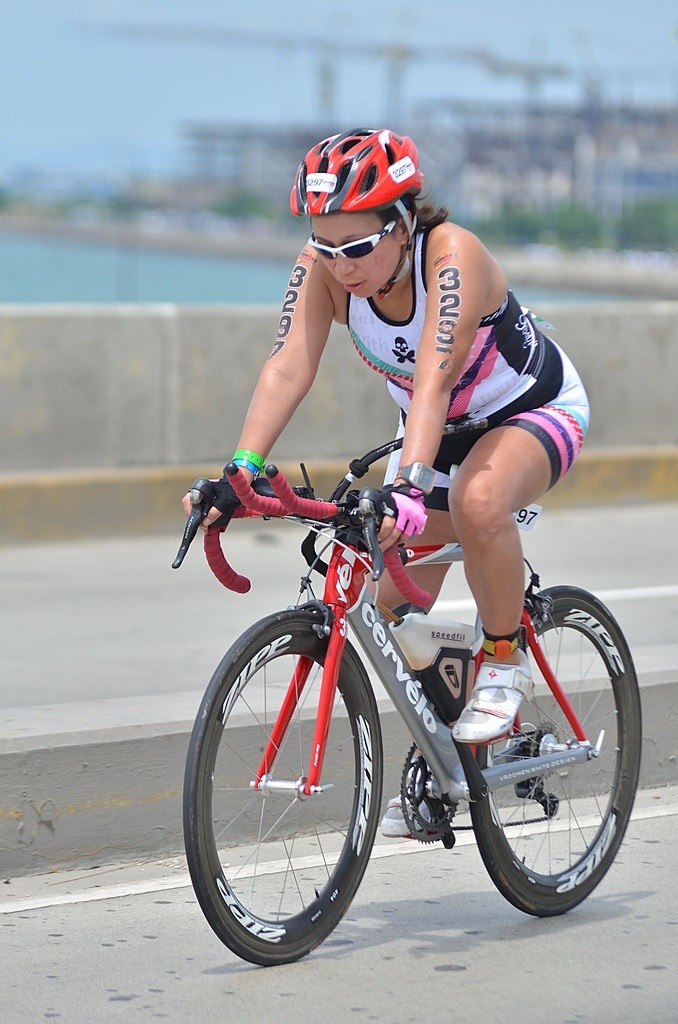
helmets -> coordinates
[290,128,423,216]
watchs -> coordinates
[394,463,438,495]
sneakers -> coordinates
[451,647,534,744]
[379,760,438,837]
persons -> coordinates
[182,127,590,837]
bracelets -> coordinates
[231,448,266,481]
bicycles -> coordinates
[172,429,643,967]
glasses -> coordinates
[307,220,397,260]
